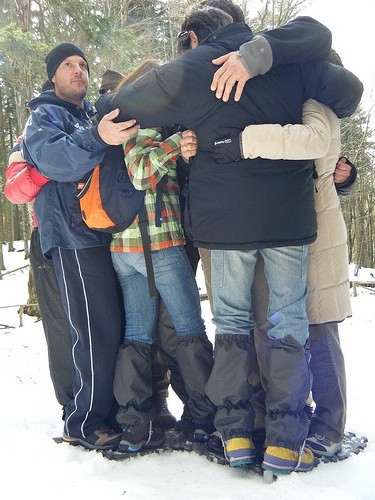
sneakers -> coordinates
[119,424,156,452]
[261,445,314,473]
[154,404,176,425]
[62,426,119,450]
[306,433,342,457]
[223,437,263,466]
[193,428,209,439]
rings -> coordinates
[184,145,188,152]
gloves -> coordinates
[211,133,242,163]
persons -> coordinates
[5,0,364,476]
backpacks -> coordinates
[75,141,148,233]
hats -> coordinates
[100,70,125,86]
[44,42,89,88]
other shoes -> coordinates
[164,420,191,441]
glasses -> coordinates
[99,85,116,94]
